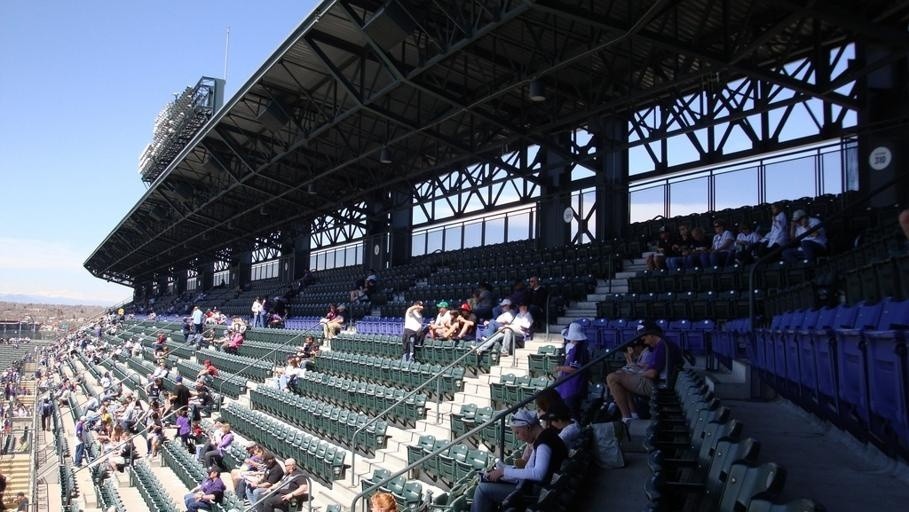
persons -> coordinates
[898,208,909,240]
[0,203,828,512]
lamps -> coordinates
[528,76,547,102]
[380,149,393,164]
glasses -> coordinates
[511,416,527,423]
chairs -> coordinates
[0,191,908,511]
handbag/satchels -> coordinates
[589,420,632,470]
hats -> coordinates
[510,407,536,425]
[437,299,511,313]
[175,360,296,474]
[792,209,806,221]
[561,322,587,341]
